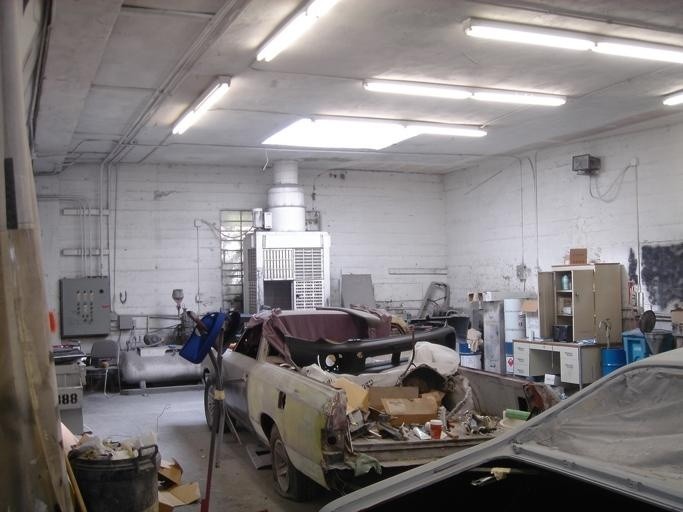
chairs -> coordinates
[83,339,123,399]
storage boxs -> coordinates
[336,377,441,428]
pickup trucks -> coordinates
[202,301,556,498]
[316,347,683,512]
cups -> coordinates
[430,420,442,439]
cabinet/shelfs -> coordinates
[511,263,624,392]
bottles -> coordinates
[561,274,570,290]
[528,329,533,341]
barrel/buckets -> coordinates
[503,298,524,343]
[601,346,624,376]
